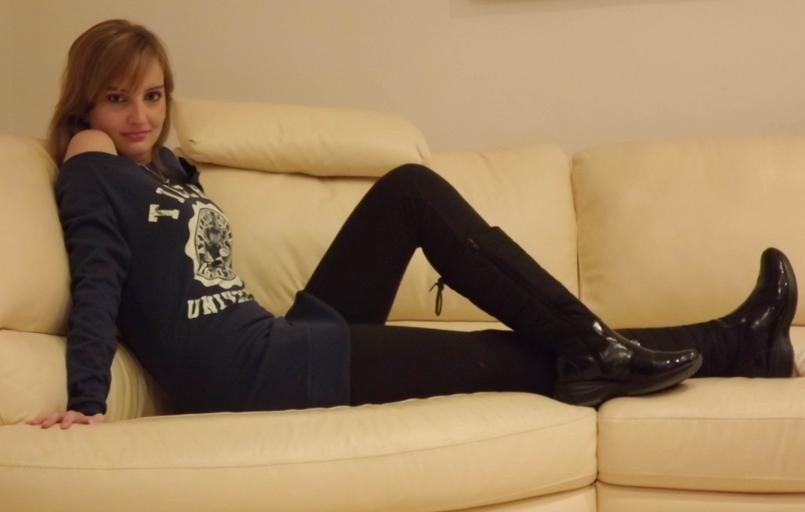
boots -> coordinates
[615,248,798,379]
[428,226,702,407]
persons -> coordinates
[27,19,798,430]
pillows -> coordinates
[156,95,427,178]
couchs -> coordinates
[0,140,805,510]
